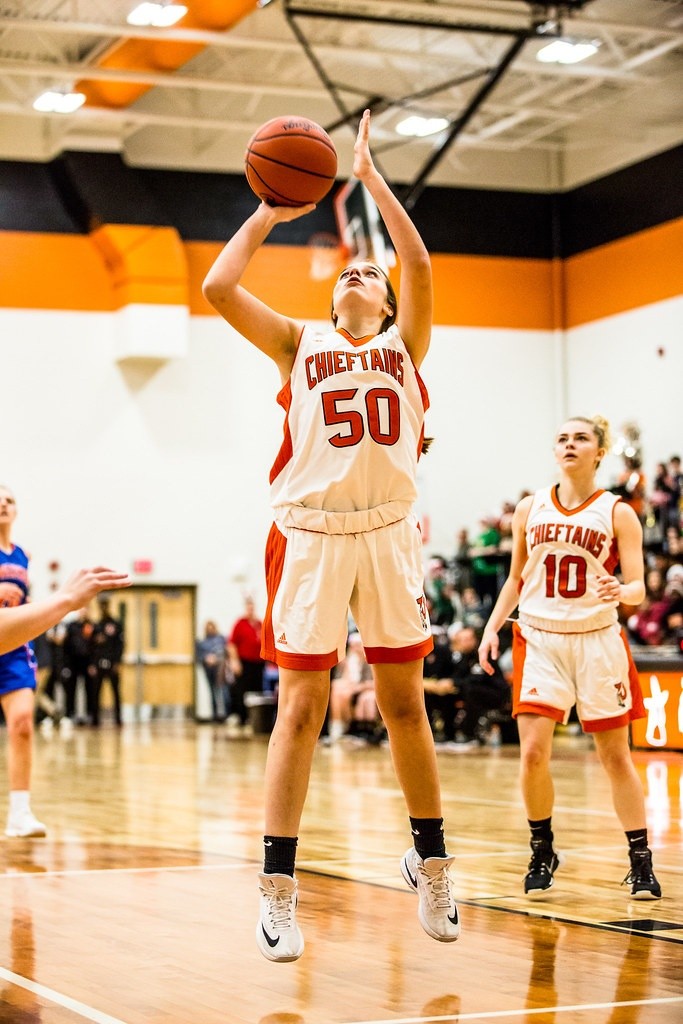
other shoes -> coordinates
[34,711,125,731]
[210,707,515,751]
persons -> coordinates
[199,108,461,964]
[227,596,260,731]
[478,416,664,903]
[0,484,45,838]
[0,568,130,655]
[92,599,124,729]
[195,622,229,724]
[260,424,683,743]
[59,617,95,725]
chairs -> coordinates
[260,487,682,750]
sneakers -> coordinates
[623,847,662,900]
[250,864,308,964]
[399,844,466,942]
[4,809,48,841]
[523,845,561,898]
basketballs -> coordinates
[244,114,340,207]
